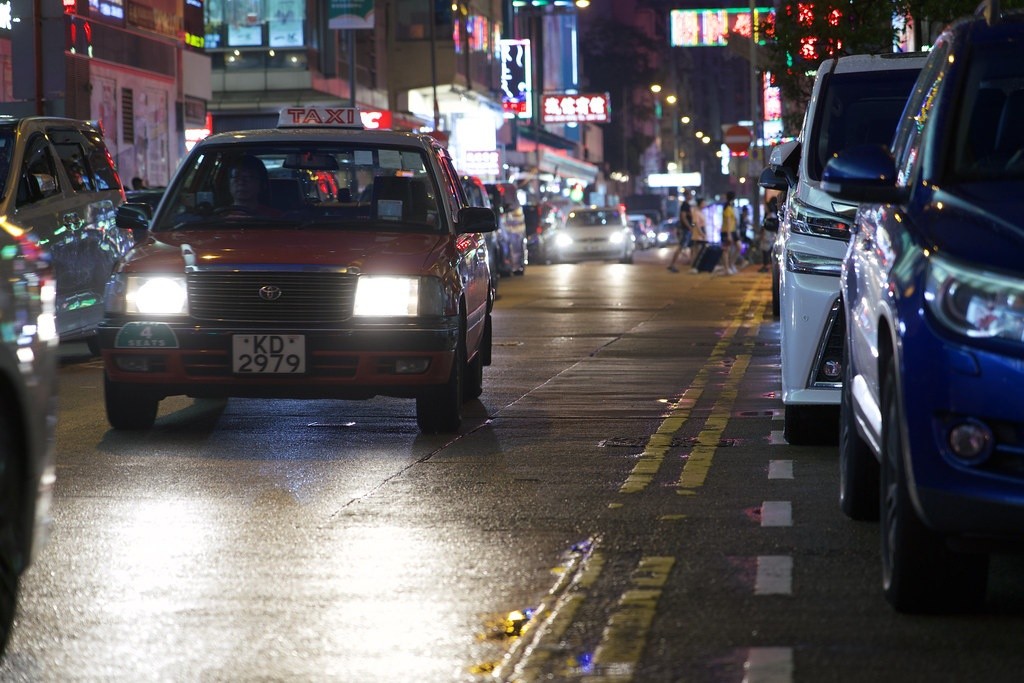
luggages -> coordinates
[690,242,730,272]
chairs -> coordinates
[969,85,1024,154]
[352,175,427,222]
[268,178,309,216]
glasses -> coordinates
[232,175,262,182]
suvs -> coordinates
[816,1,1024,612]
[757,48,942,447]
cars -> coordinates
[91,109,503,435]
[361,174,684,281]
[551,204,637,262]
[0,113,129,355]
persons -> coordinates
[205,154,284,220]
[735,205,760,269]
[123,177,148,193]
[758,196,779,273]
[687,197,711,268]
[667,190,697,274]
[719,190,738,275]
